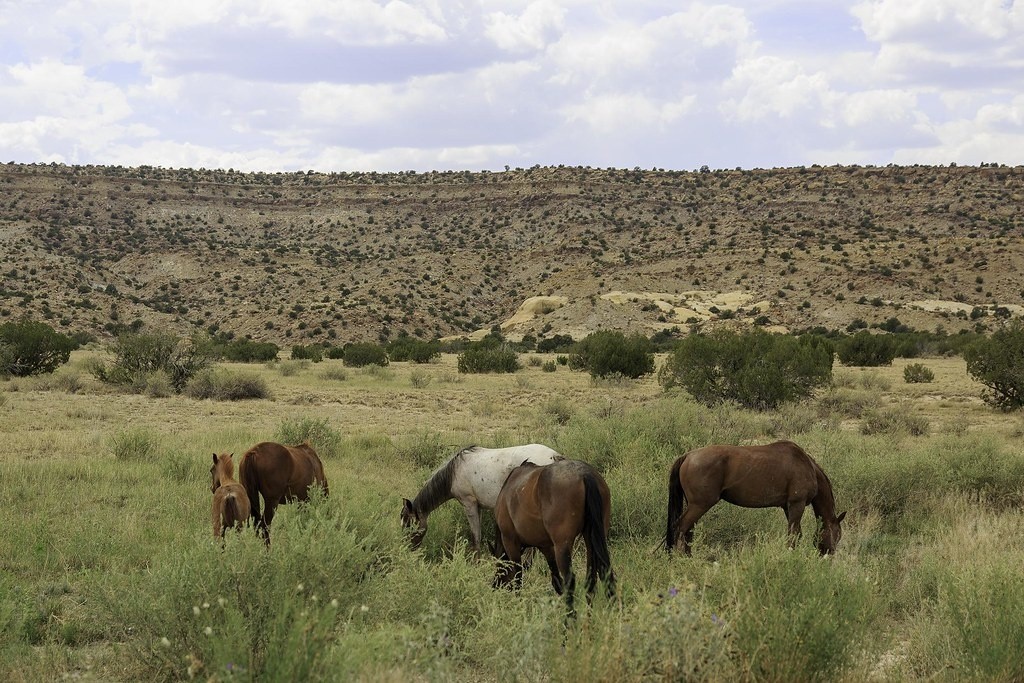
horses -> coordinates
[665,439,847,556]
[400,444,617,652]
[209,440,330,554]
